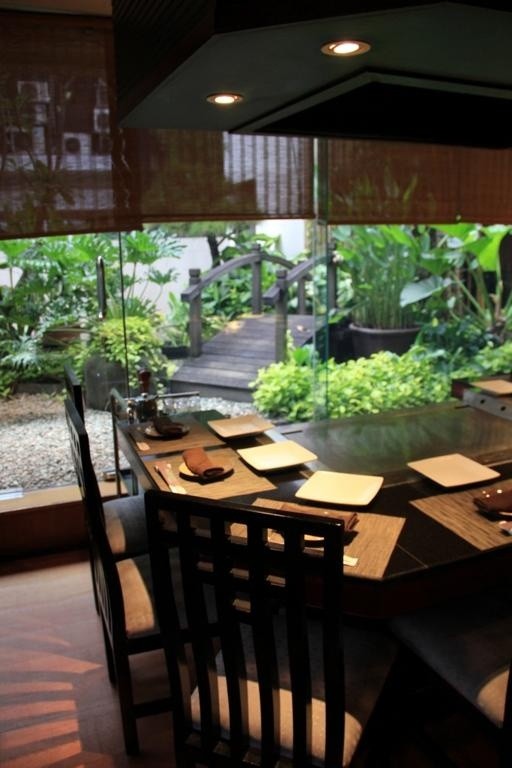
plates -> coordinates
[405,452,502,490]
[143,423,190,441]
[300,534,324,543]
[470,378,512,396]
[294,469,387,508]
[206,412,274,440]
[237,437,319,472]
[481,487,512,518]
[179,456,234,479]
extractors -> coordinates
[106,0,512,153]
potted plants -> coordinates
[350,246,424,359]
